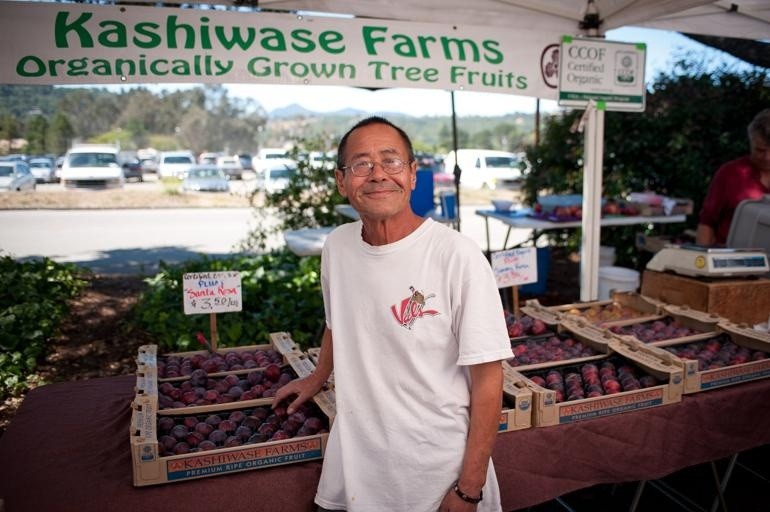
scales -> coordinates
[645,244,770,278]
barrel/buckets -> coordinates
[438,190,454,219]
[597,265,641,301]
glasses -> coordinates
[338,158,413,176]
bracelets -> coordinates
[454,479,483,504]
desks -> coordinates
[475,209,686,313]
[0,307,770,512]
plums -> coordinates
[157,349,328,458]
[504,303,767,402]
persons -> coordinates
[271,117,515,512]
[694,110,770,247]
